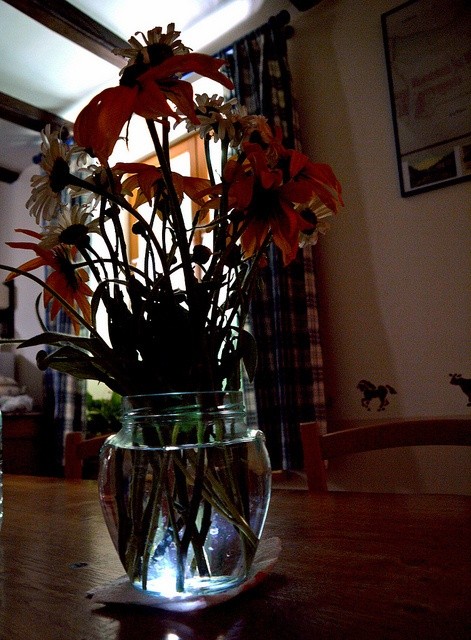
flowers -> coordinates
[0,21,347,587]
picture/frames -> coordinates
[379,1,470,197]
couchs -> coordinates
[0,337,52,467]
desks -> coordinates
[2,466,467,631]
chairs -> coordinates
[299,417,469,495]
[62,425,141,478]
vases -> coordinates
[97,391,275,610]
